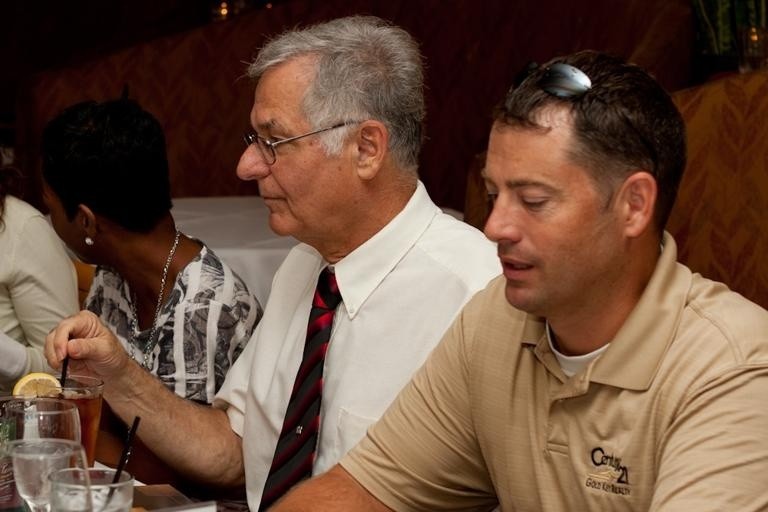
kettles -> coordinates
[0,441,93,511]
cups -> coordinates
[33,373,107,471]
[46,467,133,511]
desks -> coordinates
[166,194,302,314]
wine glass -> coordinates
[0,401,82,443]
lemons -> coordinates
[13,372,61,399]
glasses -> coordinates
[243,120,362,165]
[510,62,659,174]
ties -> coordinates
[259,267,344,512]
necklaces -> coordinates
[113,229,181,467]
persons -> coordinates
[20,96,265,407]
[256,54,767,512]
[43,14,505,510]
[0,126,83,401]
